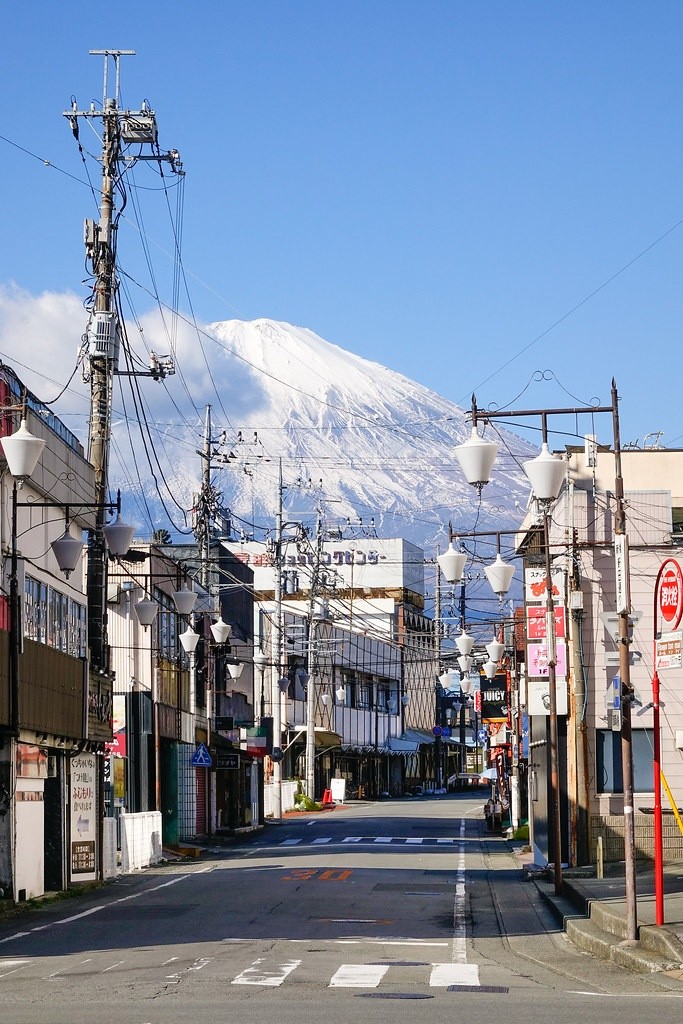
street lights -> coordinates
[433,504,566,898]
[451,368,642,945]
[9,472,136,738]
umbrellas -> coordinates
[481,767,508,779]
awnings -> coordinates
[408,729,465,747]
